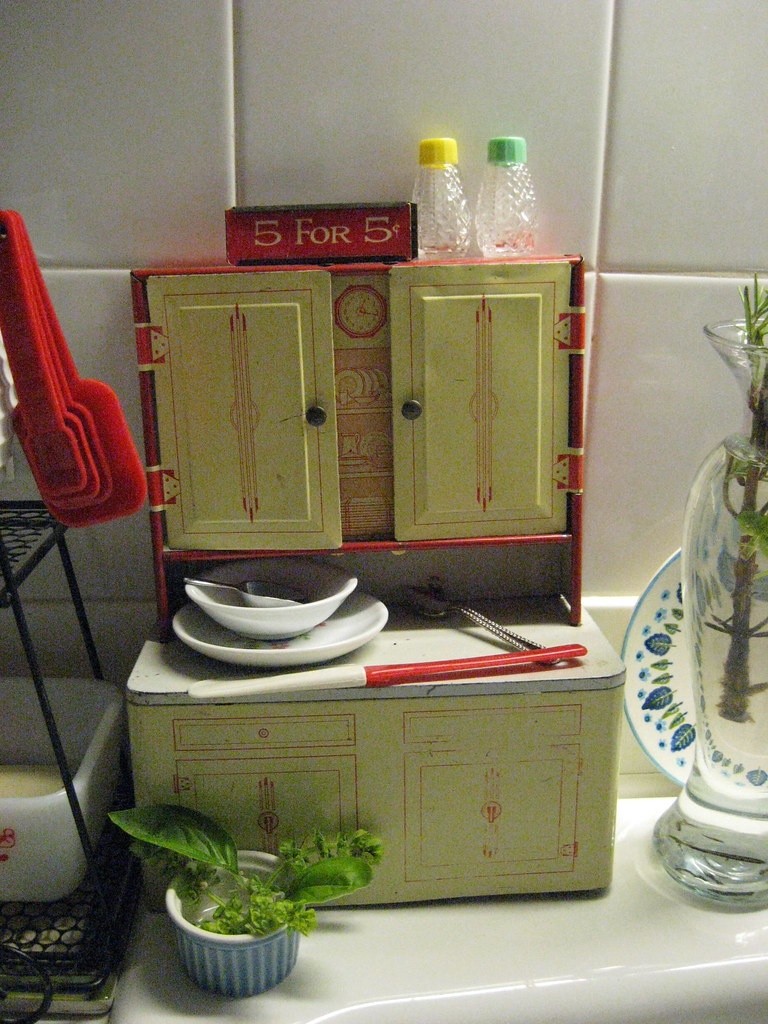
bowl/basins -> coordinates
[185,558,358,639]
[0,676,122,902]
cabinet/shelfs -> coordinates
[120,254,626,904]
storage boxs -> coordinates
[0,676,120,898]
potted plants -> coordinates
[110,805,384,999]
[621,270,766,911]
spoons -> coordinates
[406,588,559,666]
[183,576,306,608]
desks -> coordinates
[110,798,768,1023]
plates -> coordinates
[173,590,390,666]
[622,547,696,786]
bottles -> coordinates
[412,138,473,260]
[475,136,536,258]
